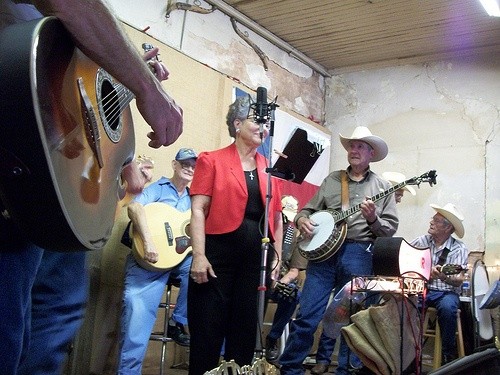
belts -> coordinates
[344,239,374,244]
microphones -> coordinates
[255,87,268,139]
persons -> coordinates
[116,149,198,375]
[264,126,417,375]
[410,203,468,365]
[0,0,183,375]
[186,96,284,375]
[420,257,429,278]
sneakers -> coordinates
[167,319,190,347]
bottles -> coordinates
[462,273,471,297]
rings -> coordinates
[193,277,196,279]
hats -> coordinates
[430,203,464,238]
[281,196,298,222]
[175,148,198,160]
[382,172,416,195]
[340,126,388,163]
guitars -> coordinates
[296,168,438,263]
[132,201,192,273]
[435,263,470,279]
[0,13,162,253]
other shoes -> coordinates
[311,363,328,375]
[266,335,281,361]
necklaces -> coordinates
[242,159,255,180]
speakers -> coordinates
[427,346,500,375]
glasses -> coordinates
[178,160,195,170]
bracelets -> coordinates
[442,275,450,283]
[273,257,283,263]
[366,215,377,226]
